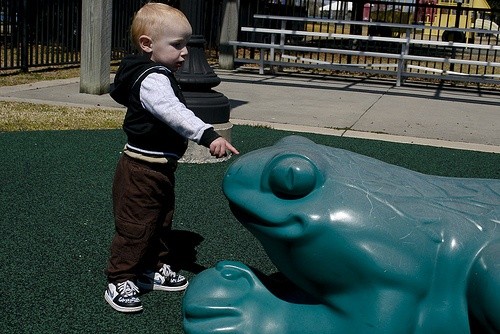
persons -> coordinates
[104,3,240,313]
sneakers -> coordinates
[137,264,189,291]
[104,280,143,311]
